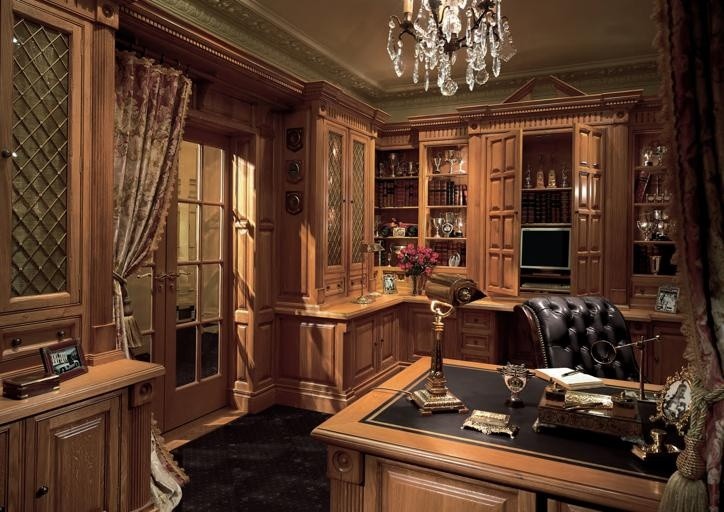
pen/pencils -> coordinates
[562,371,578,377]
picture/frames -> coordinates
[41,337,88,382]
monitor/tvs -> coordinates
[519,227,571,271]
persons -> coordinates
[664,384,687,417]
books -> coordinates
[634,170,667,203]
[374,178,468,207]
[533,367,604,392]
[520,192,573,224]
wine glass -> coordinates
[385,152,402,178]
[432,149,468,174]
[636,220,658,241]
[431,217,446,238]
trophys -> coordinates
[637,209,676,241]
[430,211,465,238]
[522,160,570,187]
[378,148,466,178]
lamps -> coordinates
[386,0,519,97]
[406,273,478,416]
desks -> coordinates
[310,355,687,512]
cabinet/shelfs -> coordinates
[373,88,680,310]
[403,304,456,367]
[456,308,507,364]
[0,357,165,512]
[276,303,402,415]
[273,81,392,310]
[652,323,686,384]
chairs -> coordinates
[515,295,649,384]
